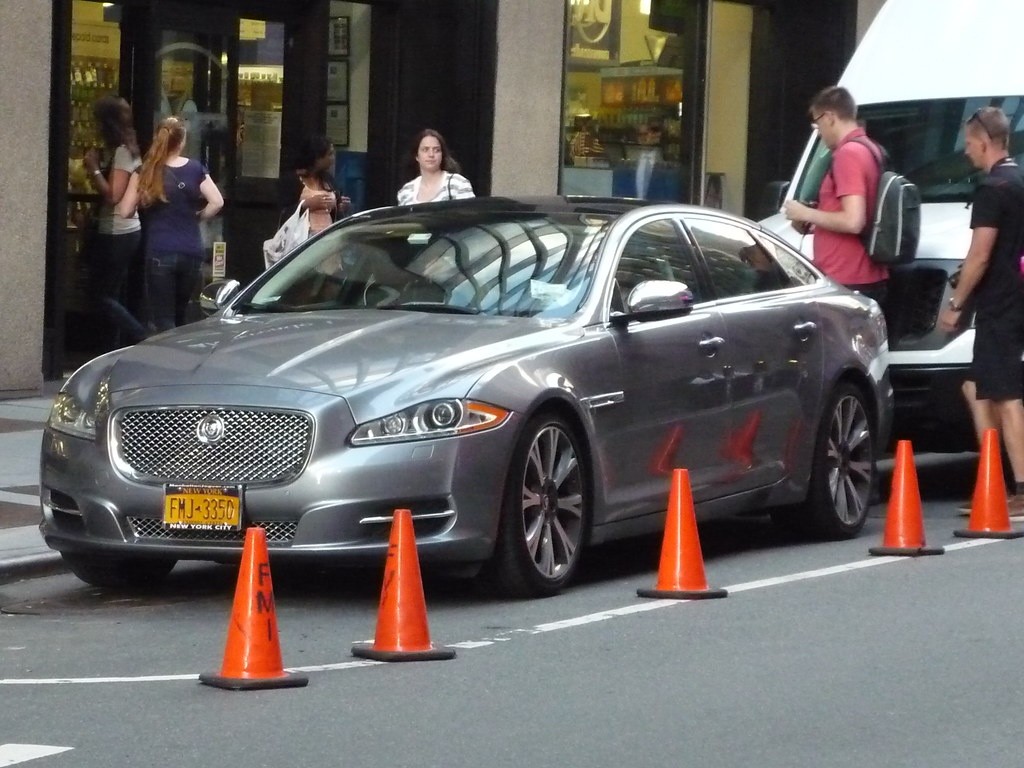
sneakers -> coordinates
[1007,494,1024,522]
[955,502,973,515]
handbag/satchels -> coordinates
[193,193,208,211]
[83,216,98,262]
[263,199,311,270]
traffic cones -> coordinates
[350,508,456,661]
[637,468,728,601]
[647,419,684,475]
[868,439,945,556]
[716,407,761,468]
[953,427,1024,540]
[198,527,308,689]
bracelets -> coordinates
[90,170,102,179]
[946,297,962,312]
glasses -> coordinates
[967,108,993,140]
[811,111,834,128]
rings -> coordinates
[323,197,326,200]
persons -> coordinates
[273,136,351,265]
[787,88,891,356]
[396,128,478,206]
[739,244,793,292]
[80,97,224,349]
[937,106,1024,521]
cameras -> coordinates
[950,264,964,289]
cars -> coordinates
[39,196,893,597]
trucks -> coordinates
[755,0,1023,449]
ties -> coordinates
[641,157,653,199]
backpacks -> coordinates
[828,137,921,267]
